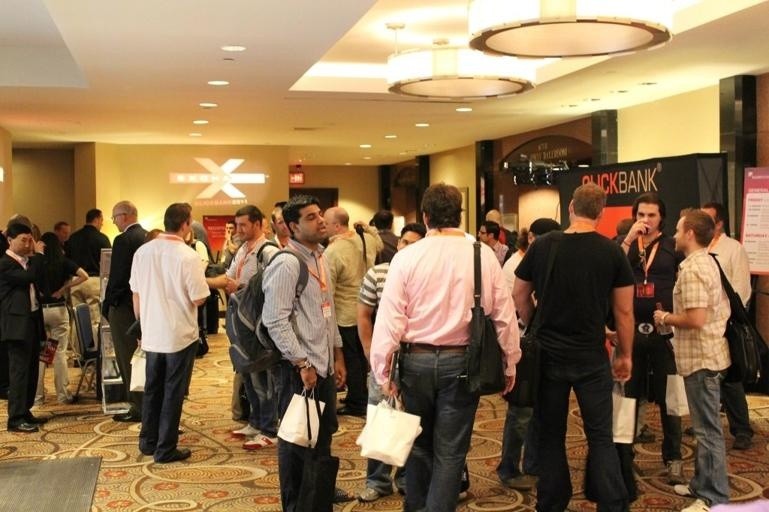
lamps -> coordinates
[385,0,672,102]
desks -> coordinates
[0,454,102,512]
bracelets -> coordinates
[621,239,631,247]
[660,312,669,327]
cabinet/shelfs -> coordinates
[100,325,131,416]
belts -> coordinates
[632,322,658,336]
[41,303,67,308]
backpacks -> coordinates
[225,265,281,370]
[724,292,769,398]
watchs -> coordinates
[299,360,312,369]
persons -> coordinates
[129,202,210,464]
[358,222,427,502]
[615,192,689,485]
[261,199,346,512]
[700,202,757,449]
[0,201,560,451]
[512,181,636,512]
[370,182,521,511]
[655,208,732,512]
[1,222,48,431]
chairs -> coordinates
[72,303,100,400]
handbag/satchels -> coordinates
[354,392,425,470]
[611,379,638,446]
[275,386,327,450]
[128,341,147,393]
[463,306,507,398]
[664,373,690,418]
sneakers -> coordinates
[502,476,535,491]
[331,486,381,503]
[729,433,750,453]
[35,398,44,405]
[242,434,277,450]
[57,393,73,403]
[667,459,686,485]
[681,498,709,512]
[674,484,691,496]
[230,424,263,439]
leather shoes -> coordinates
[336,397,366,417]
[7,417,48,434]
[112,412,140,423]
[140,448,191,463]
